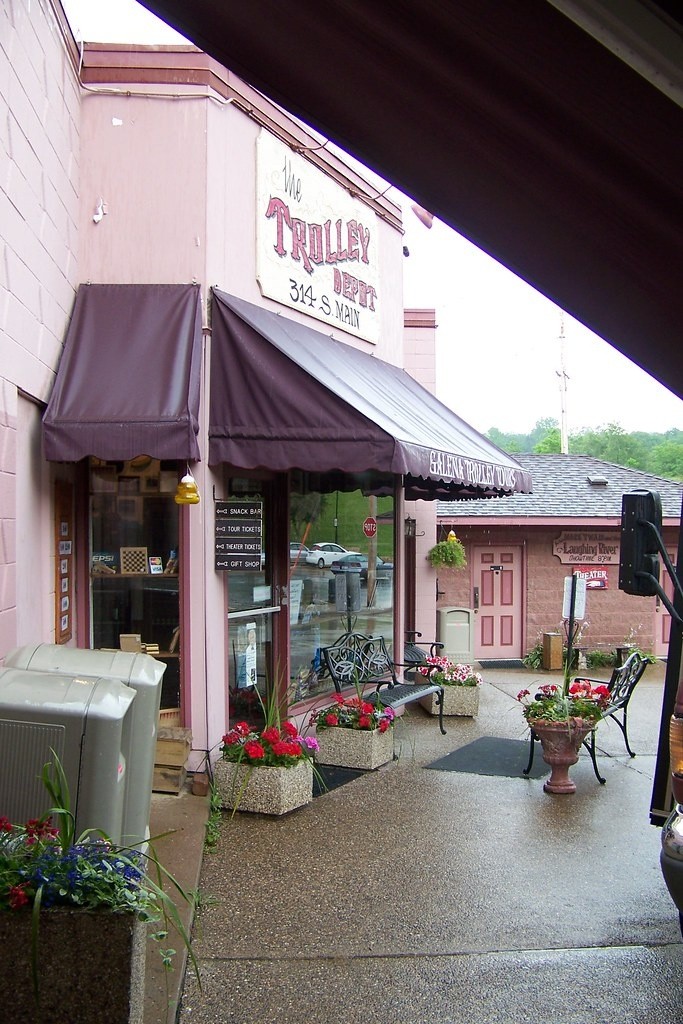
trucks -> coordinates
[619,487,683,935]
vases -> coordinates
[313,725,394,771]
[528,722,596,795]
[0,905,134,1024]
[415,672,479,717]
[213,757,314,817]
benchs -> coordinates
[304,633,373,682]
[522,653,650,786]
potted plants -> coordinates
[425,537,468,574]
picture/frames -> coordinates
[118,499,135,515]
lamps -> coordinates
[447,521,457,541]
[174,460,201,505]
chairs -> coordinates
[324,632,447,762]
[404,631,448,736]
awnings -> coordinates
[209,286,534,501]
[40,282,202,465]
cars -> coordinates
[290,542,311,566]
[306,543,361,569]
[330,554,394,579]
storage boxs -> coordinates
[89,464,119,493]
[119,546,149,574]
[159,470,179,493]
[148,556,162,569]
[542,633,563,671]
[119,634,141,653]
[151,564,164,575]
[117,476,140,493]
[155,727,192,767]
[152,765,188,797]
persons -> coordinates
[246,630,256,687]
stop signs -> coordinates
[363,517,377,538]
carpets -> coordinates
[422,735,553,780]
[311,763,378,798]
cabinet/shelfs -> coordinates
[89,494,183,658]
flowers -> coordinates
[494,656,630,749]
[228,682,268,720]
[0,743,206,1024]
[303,660,417,767]
[413,654,484,687]
[190,651,330,824]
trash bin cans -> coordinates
[437,606,475,669]
[0,667,137,855]
[1,642,167,860]
